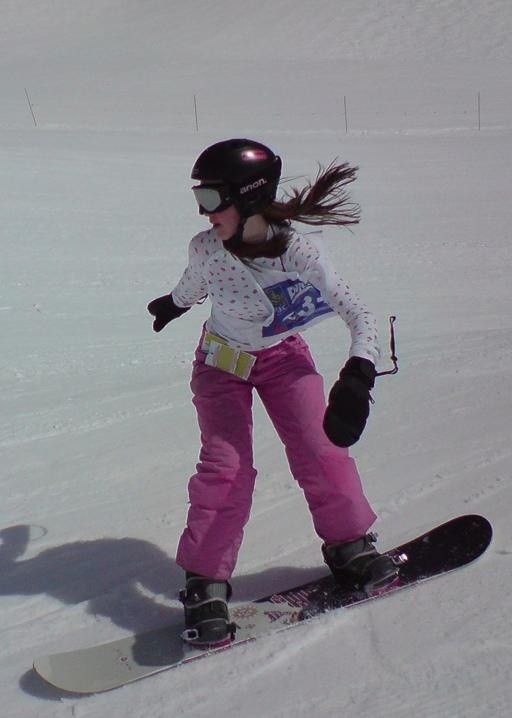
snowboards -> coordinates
[33,515,493,693]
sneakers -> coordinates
[177,572,241,649]
[318,529,403,586]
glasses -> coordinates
[192,182,239,216]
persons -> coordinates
[148,138,399,649]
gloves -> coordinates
[147,292,192,333]
[322,353,379,448]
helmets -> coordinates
[189,138,282,185]
